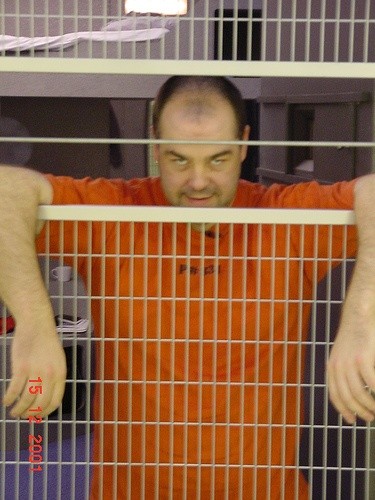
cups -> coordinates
[51,266,72,282]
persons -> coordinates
[0,76,375,500]
